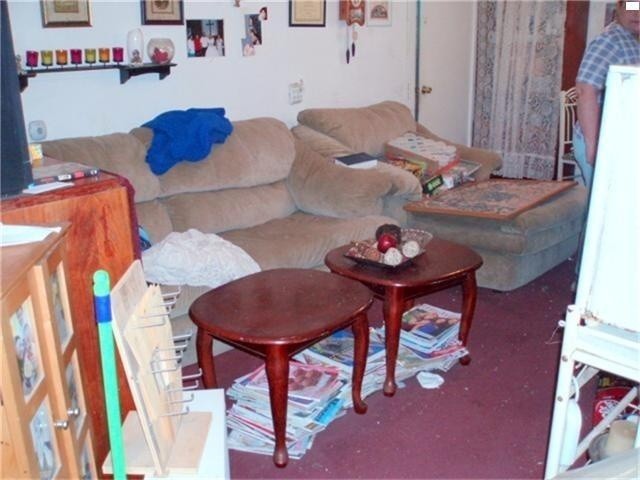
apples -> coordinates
[378,234,398,254]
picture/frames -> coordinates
[140,0,183,25]
[365,0,391,27]
[289,0,326,27]
[39,1,92,27]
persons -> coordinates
[243,26,261,56]
[571,0,640,295]
[188,33,224,57]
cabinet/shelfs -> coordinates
[0,222,100,480]
[540,306,640,480]
[1,157,146,479]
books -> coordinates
[32,161,101,185]
[223,300,469,461]
[333,152,378,169]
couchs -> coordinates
[31,117,400,369]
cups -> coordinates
[26,47,123,67]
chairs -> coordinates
[291,101,504,227]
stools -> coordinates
[403,180,587,292]
